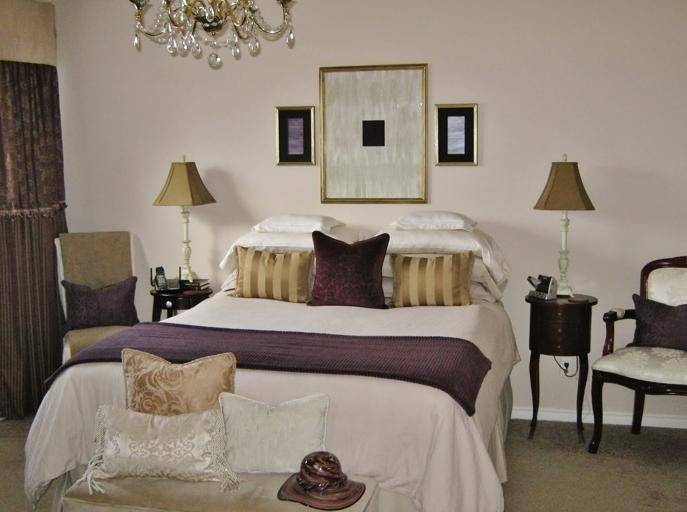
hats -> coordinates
[276,451,367,510]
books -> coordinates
[180,279,211,291]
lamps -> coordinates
[533,152,597,298]
[151,153,218,283]
[130,1,297,71]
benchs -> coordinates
[60,472,380,512]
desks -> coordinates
[524,293,599,442]
[149,286,213,323]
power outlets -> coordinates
[557,356,576,377]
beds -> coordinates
[22,289,523,512]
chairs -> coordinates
[52,230,141,367]
[587,255,687,455]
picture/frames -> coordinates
[318,62,429,203]
[273,105,317,167]
[433,102,479,167]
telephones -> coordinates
[150,265,183,294]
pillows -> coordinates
[59,275,140,336]
[119,346,236,416]
[625,292,687,355]
[219,210,508,307]
[77,405,232,480]
[214,391,328,493]
